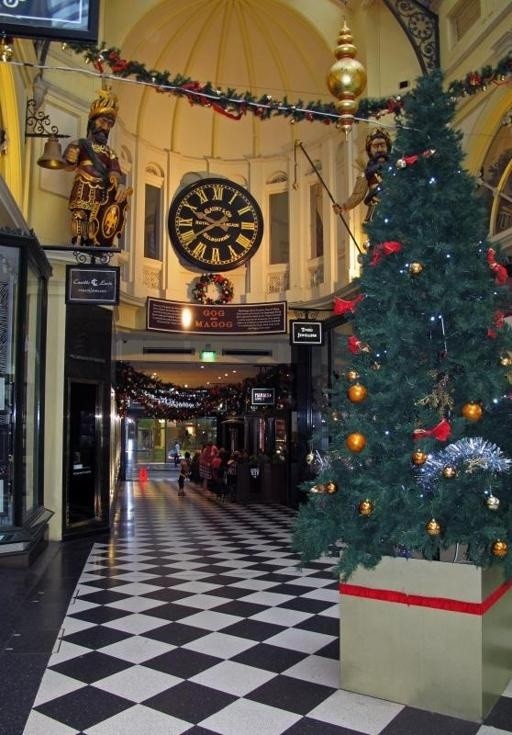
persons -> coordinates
[331,124,393,223]
[269,393,273,402]
[263,393,268,401]
[173,440,251,497]
[62,111,134,249]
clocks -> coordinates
[168,176,265,271]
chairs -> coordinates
[212,468,235,503]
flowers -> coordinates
[192,274,235,306]
